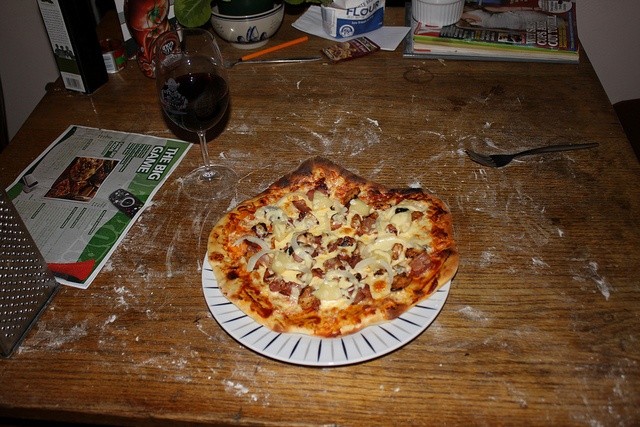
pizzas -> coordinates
[208,156,460,336]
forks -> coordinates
[464,140,604,169]
[209,33,310,68]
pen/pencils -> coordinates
[239,35,308,62]
[237,57,323,64]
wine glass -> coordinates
[154,27,238,202]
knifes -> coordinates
[202,54,326,69]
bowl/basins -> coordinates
[207,0,287,51]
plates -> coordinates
[199,246,455,368]
